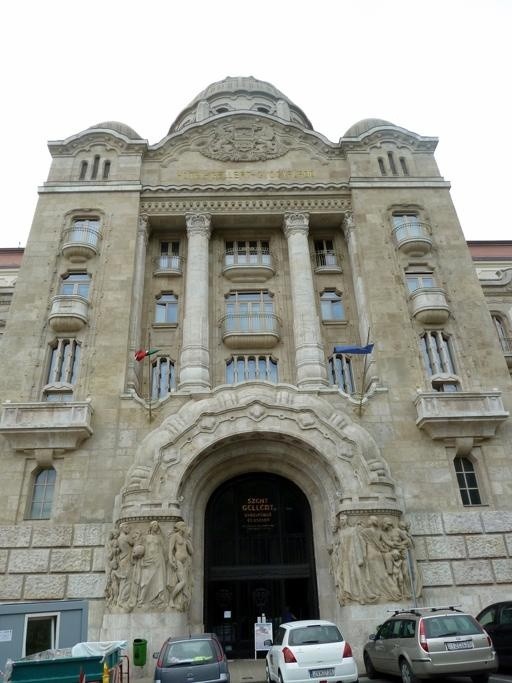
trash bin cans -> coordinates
[133,639,147,666]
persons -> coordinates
[280,605,297,624]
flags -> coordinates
[134,348,160,361]
[332,342,374,354]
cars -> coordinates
[362,604,499,683]
[153,633,231,683]
[263,619,360,683]
[476,601,512,675]
